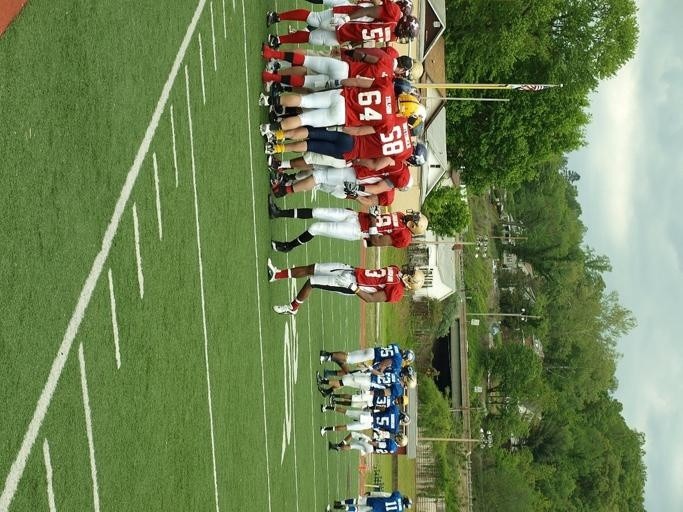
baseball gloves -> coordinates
[335,272,359,293]
